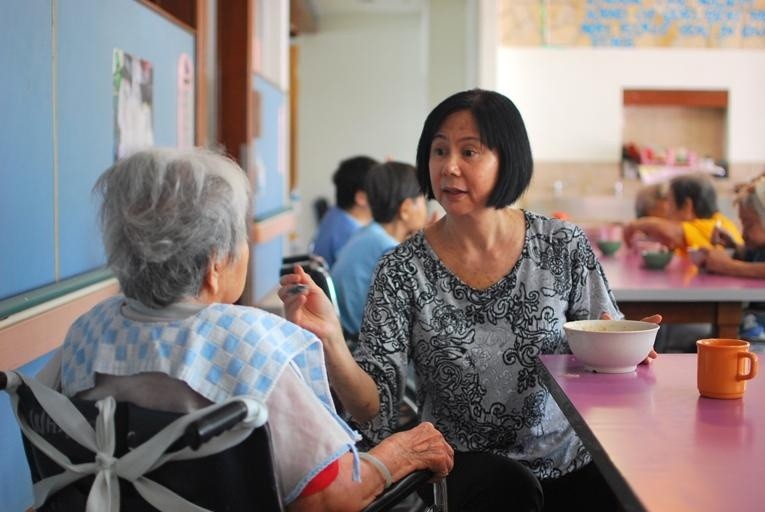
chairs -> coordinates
[2,343,448,511]
[279,256,341,316]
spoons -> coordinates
[285,281,310,296]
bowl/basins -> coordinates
[597,238,623,257]
[560,317,661,377]
[641,250,672,271]
[688,246,737,269]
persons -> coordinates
[311,153,382,272]
[56,142,545,511]
[615,166,765,357]
[326,159,431,351]
[280,87,661,511]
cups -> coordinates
[695,337,760,401]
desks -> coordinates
[585,228,765,343]
[537,352,765,510]
